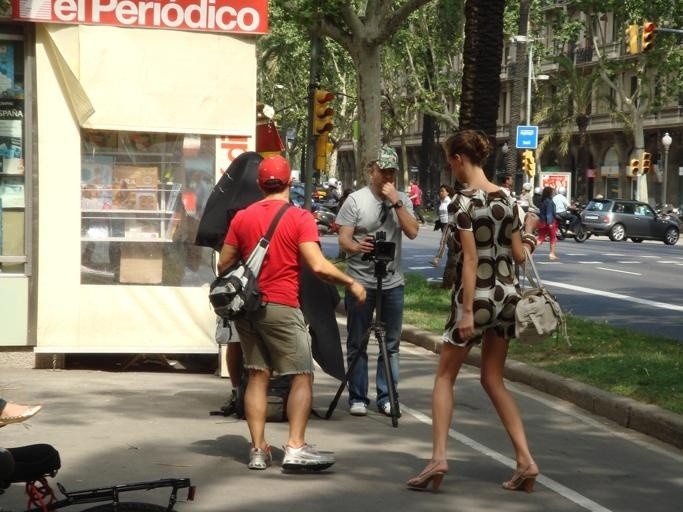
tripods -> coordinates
[326,262,401,427]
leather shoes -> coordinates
[0,405,42,429]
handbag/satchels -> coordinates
[515,284,562,345]
[237,376,289,422]
[434,220,442,232]
[209,261,262,320]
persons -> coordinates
[214,153,368,475]
[214,261,244,416]
[337,172,605,260]
[332,142,419,416]
[405,128,542,494]
[183,169,213,269]
[0,401,45,428]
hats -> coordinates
[258,154,291,190]
[367,145,399,171]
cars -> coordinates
[582,198,680,245]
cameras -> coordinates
[361,234,396,262]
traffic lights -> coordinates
[630,159,638,176]
[642,151,652,174]
[521,151,532,171]
[315,136,335,171]
[311,90,336,136]
[623,26,640,54]
[641,21,659,51]
[527,157,536,177]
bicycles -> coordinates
[0,443,198,512]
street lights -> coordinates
[660,132,672,205]
[506,34,550,194]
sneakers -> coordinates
[350,401,369,416]
[549,256,558,262]
[280,444,338,468]
[221,390,237,411]
[379,402,402,417]
[248,441,274,470]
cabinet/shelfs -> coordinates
[81,127,184,284]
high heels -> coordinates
[501,459,541,492]
[406,461,451,488]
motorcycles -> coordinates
[290,184,353,236]
[554,207,588,242]
